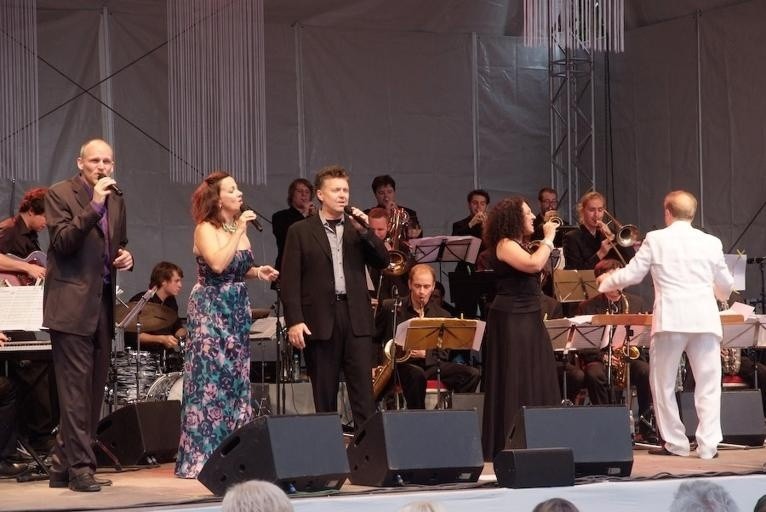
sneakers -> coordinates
[33,438,56,457]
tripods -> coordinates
[622,340,663,455]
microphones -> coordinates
[97,172,125,198]
[537,221,582,233]
[342,203,372,231]
[393,286,404,308]
[745,255,765,266]
[240,202,264,232]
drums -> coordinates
[104,351,161,404]
[146,371,183,402]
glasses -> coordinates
[542,199,558,205]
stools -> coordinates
[723,375,751,391]
[394,379,449,410]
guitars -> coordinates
[0,249,46,286]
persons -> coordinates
[123,262,187,353]
[479,197,566,460]
[0,187,57,479]
[41,139,134,492]
[595,189,733,461]
[531,499,581,512]
[359,170,457,347]
[270,178,315,294]
[401,499,446,512]
[174,172,279,478]
[451,190,491,321]
[665,480,766,511]
[222,476,293,512]
[278,167,407,488]
[529,190,660,447]
[378,266,480,410]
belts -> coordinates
[336,294,347,301]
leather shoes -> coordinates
[0,460,28,479]
[649,448,679,456]
[694,444,718,458]
[69,474,101,492]
[5,449,34,464]
[49,476,112,488]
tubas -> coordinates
[381,199,410,276]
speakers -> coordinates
[352,410,484,488]
[492,446,576,485]
[675,387,766,443]
[197,410,351,497]
[94,400,182,466]
[510,404,634,480]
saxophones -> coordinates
[616,288,641,391]
[373,300,425,400]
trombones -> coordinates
[599,209,641,268]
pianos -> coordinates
[0,341,53,353]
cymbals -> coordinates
[116,300,178,332]
